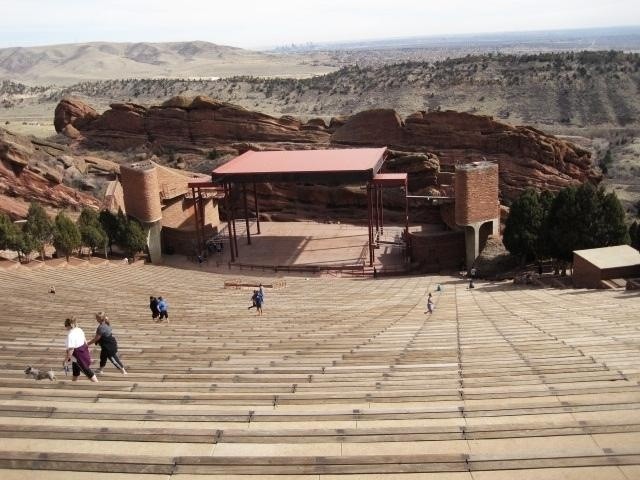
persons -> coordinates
[475,267,481,280]
[248,290,257,309]
[470,267,476,280]
[149,297,160,320]
[88,310,130,377]
[47,286,55,295]
[187,240,224,265]
[374,267,377,279]
[156,297,169,323]
[63,315,99,383]
[423,292,435,316]
[254,291,264,314]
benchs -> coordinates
[0,262,640,480]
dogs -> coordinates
[24,366,57,381]
